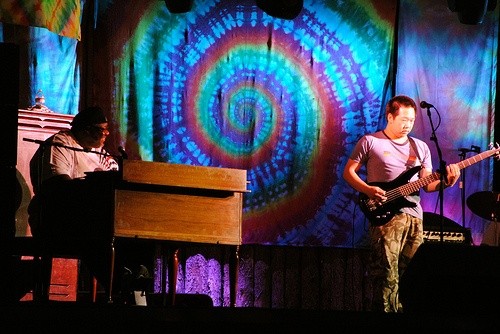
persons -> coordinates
[28,107,119,238]
[343,96,461,313]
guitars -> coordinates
[357,142,500,228]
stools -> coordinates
[12,236,97,304]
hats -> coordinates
[70,106,109,127]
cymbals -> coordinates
[466,191,500,223]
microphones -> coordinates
[419,101,434,108]
[119,145,130,161]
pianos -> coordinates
[78,157,254,309]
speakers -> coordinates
[399,241,500,313]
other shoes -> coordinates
[132,264,150,279]
